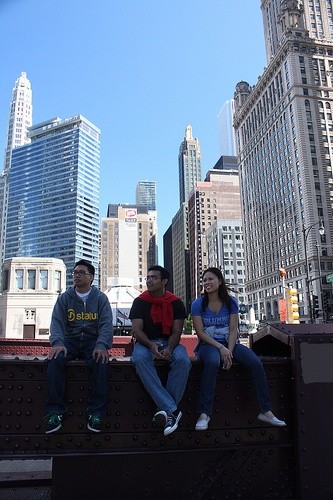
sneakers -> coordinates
[87,415,102,432]
[43,412,62,433]
[153,411,183,435]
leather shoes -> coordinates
[195,413,210,430]
[258,411,286,426]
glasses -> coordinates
[71,270,91,276]
[145,277,160,282]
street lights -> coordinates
[302,219,324,322]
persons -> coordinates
[192,267,287,430]
[129,265,189,436]
[37,259,114,435]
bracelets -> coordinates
[218,346,224,350]
